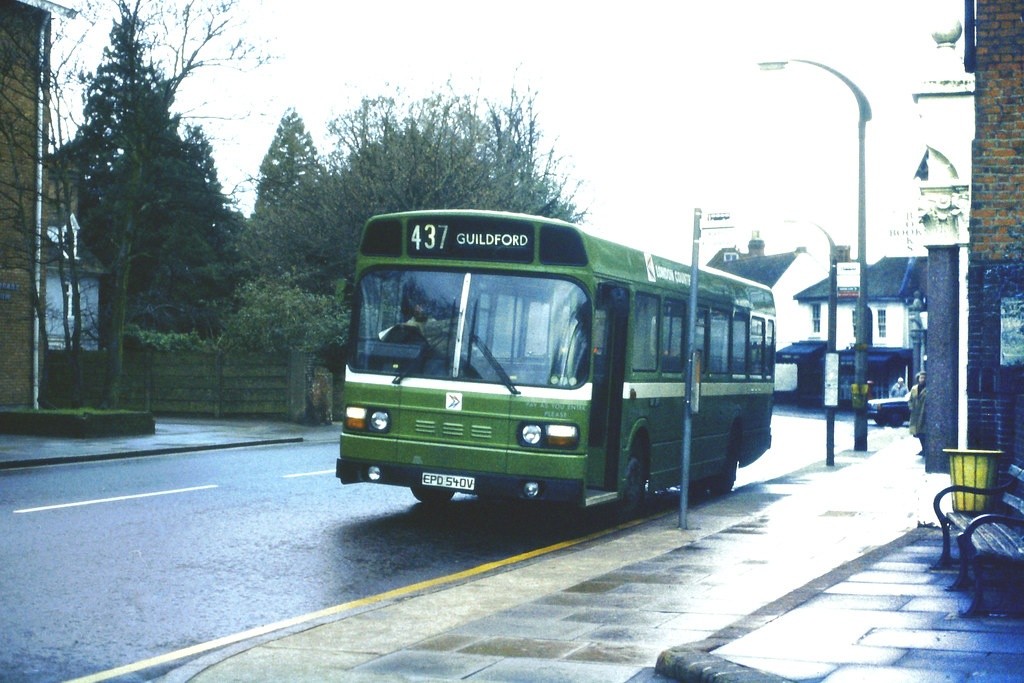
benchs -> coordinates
[928,463,1024,619]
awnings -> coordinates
[775,342,826,364]
[839,347,903,367]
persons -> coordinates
[407,295,447,347]
[889,377,909,399]
[908,371,927,456]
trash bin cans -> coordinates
[943,447,1006,515]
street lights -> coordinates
[758,55,872,451]
[782,213,840,467]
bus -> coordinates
[336,208,776,519]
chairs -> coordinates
[661,354,772,375]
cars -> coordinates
[867,390,912,427]
